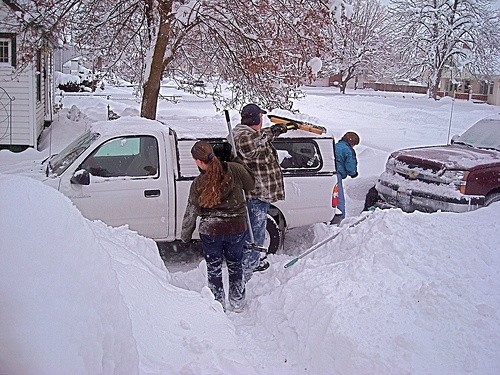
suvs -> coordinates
[362,117,500,214]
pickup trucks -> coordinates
[45,119,339,254]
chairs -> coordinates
[139,148,157,176]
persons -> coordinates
[232,103,299,272]
[334,132,360,218]
[180,140,257,314]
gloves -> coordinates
[351,172,358,178]
[270,123,287,137]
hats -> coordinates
[241,104,267,118]
[342,132,359,145]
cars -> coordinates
[180,76,204,86]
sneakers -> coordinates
[253,261,269,272]
[233,300,247,313]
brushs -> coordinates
[223,109,268,254]
[260,113,326,142]
[284,201,396,270]
